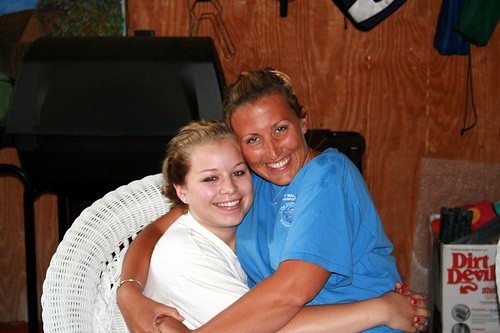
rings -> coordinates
[154,322,158,325]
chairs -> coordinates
[39,172,173,333]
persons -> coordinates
[116,68,410,333]
[142,119,429,333]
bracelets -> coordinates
[117,278,144,292]
